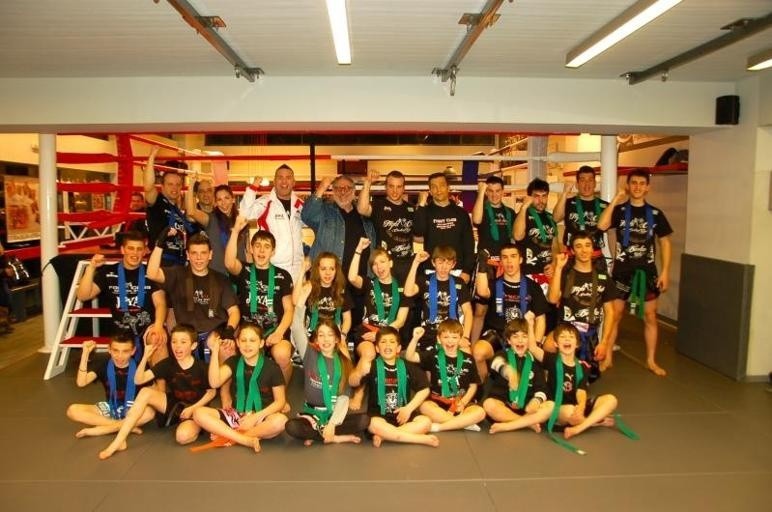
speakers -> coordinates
[715,95,740,124]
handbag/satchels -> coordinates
[6,256,30,285]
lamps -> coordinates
[745,45,771,74]
[564,0,687,70]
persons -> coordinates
[98,322,215,459]
[347,326,439,448]
[67,330,155,438]
[524,311,618,441]
[132,146,304,378]
[481,318,557,435]
[406,319,487,434]
[292,171,476,352]
[75,231,168,368]
[192,324,288,453]
[472,166,672,376]
[283,281,370,447]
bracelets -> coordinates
[79,365,89,373]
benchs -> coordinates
[9,272,41,321]
